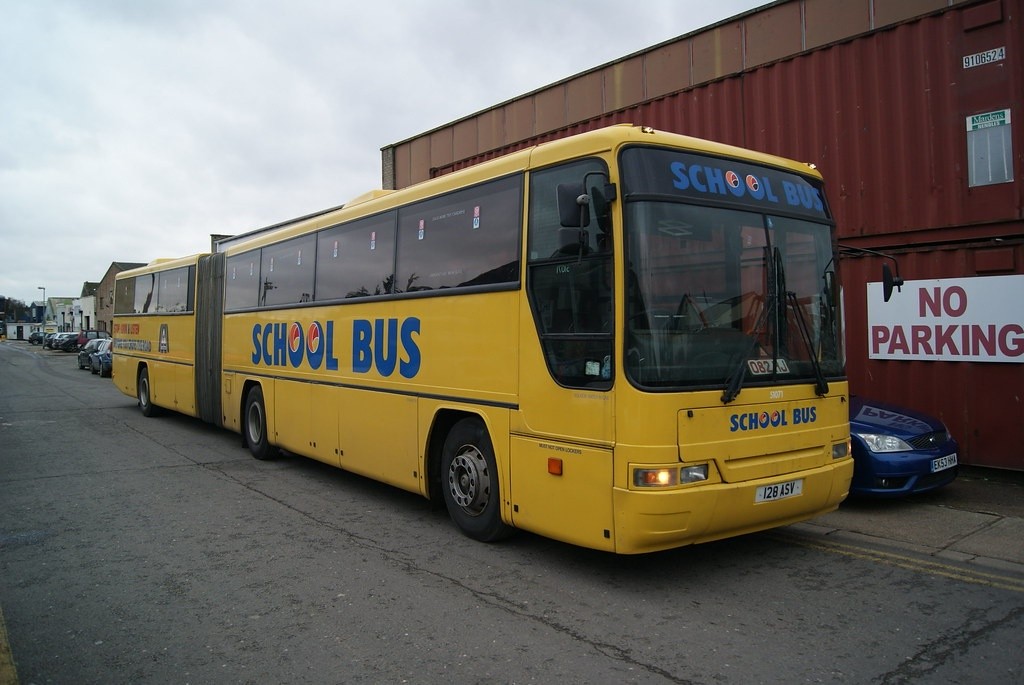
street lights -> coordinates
[38,287,46,349]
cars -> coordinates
[847,392,960,496]
[28,326,116,379]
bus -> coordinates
[110,123,903,554]
